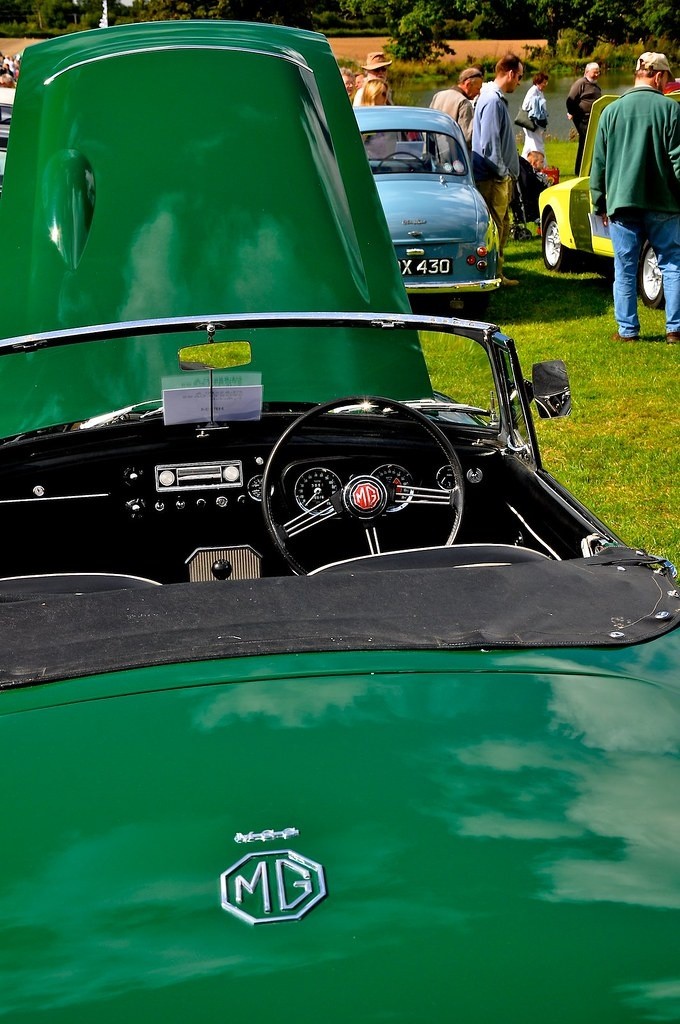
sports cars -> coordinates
[0,13,680,1024]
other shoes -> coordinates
[610,333,638,344]
[666,331,680,344]
[497,274,519,286]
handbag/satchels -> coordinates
[514,93,541,132]
[541,165,559,185]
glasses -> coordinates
[380,91,386,97]
[375,68,386,73]
[463,73,483,82]
[514,70,523,81]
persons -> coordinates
[339,51,395,106]
[0,51,21,89]
[566,62,601,176]
[588,52,679,345]
[429,54,560,286]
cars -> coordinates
[537,94,666,309]
[353,106,503,317]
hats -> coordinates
[636,52,676,82]
[361,52,392,70]
